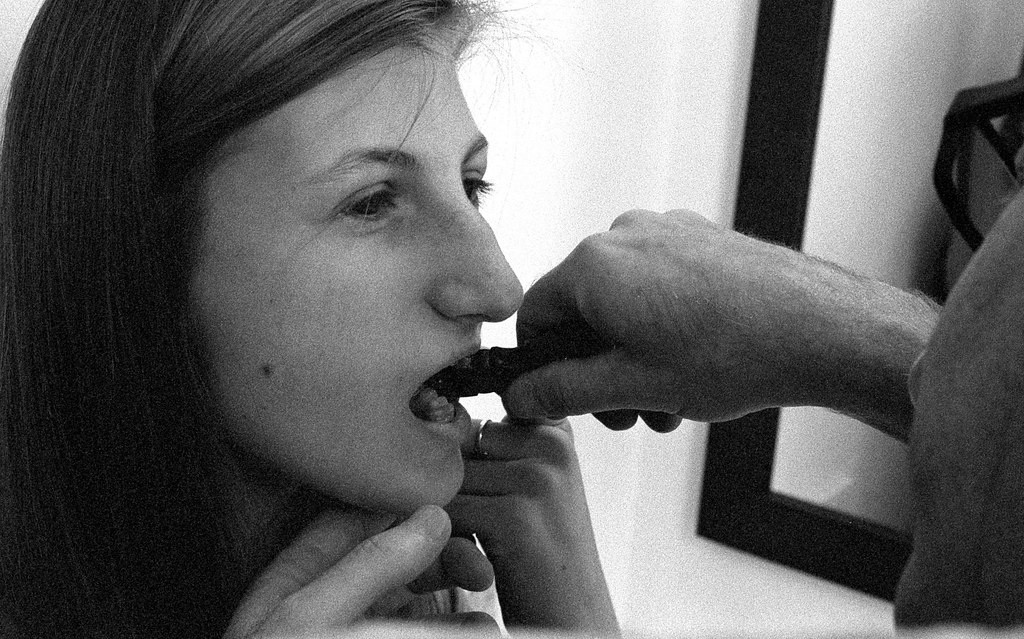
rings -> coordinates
[475,419,490,457]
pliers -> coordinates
[434,320,601,397]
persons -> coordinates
[0,0,618,639]
[227,55,1024,639]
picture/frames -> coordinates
[693,1,1023,605]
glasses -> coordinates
[933,74,1024,251]
